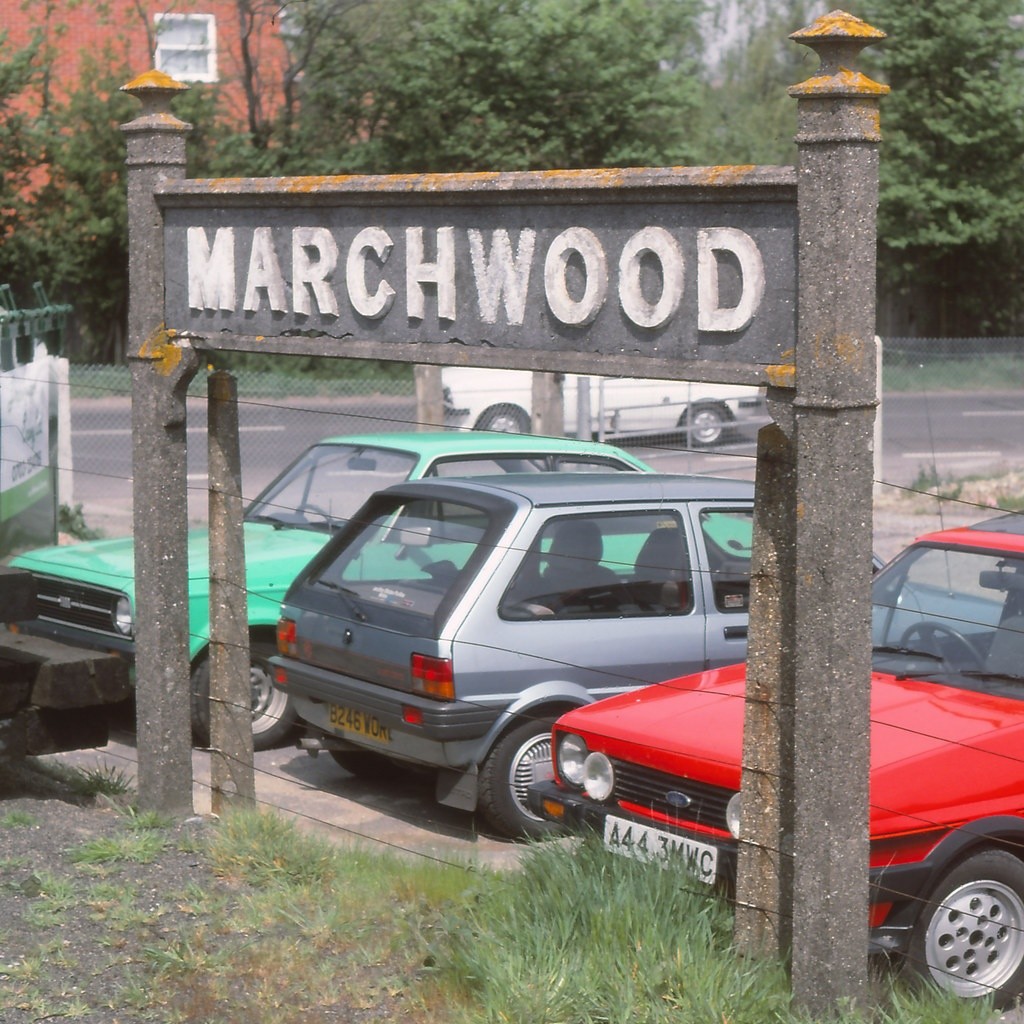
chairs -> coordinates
[636,528,725,602]
[541,519,621,598]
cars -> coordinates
[1,429,652,754]
[441,365,760,451]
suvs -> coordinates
[264,473,1003,841]
[529,512,1022,1007]
